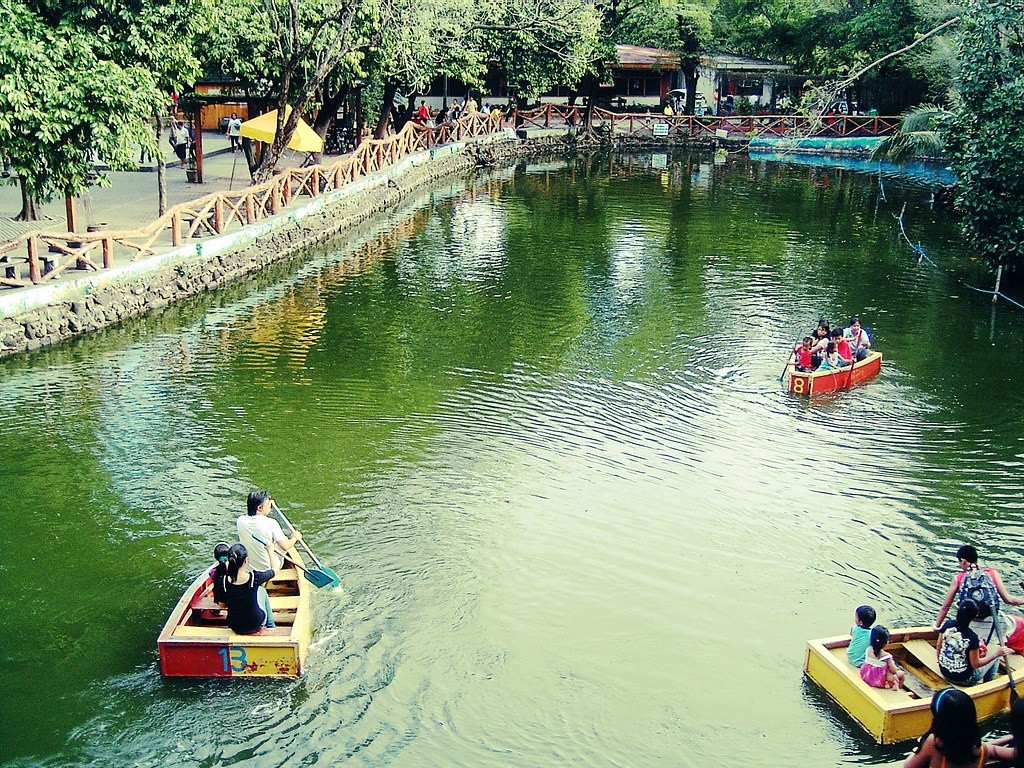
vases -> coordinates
[185,168,198,183]
[87,222,108,232]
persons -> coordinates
[932,546,1024,656]
[209,543,230,582]
[238,491,302,585]
[646,109,651,124]
[436,107,449,125]
[675,95,684,115]
[225,113,244,154]
[816,342,848,372]
[169,116,197,169]
[449,95,502,120]
[831,328,853,365]
[817,106,844,133]
[713,89,718,115]
[216,539,280,635]
[903,687,1024,768]
[843,318,871,361]
[664,104,674,124]
[937,600,1008,687]
[860,626,905,689]
[784,319,831,372]
[417,100,431,125]
[846,606,877,667]
[387,112,394,136]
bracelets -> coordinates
[934,623,942,630]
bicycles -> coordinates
[324,126,356,155]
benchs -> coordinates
[38,242,99,280]
[0,257,30,279]
[191,596,300,610]
[901,640,983,691]
[171,624,292,637]
[829,648,914,705]
[268,568,298,581]
[998,653,1024,672]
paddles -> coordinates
[251,504,342,588]
[776,329,802,381]
[844,330,863,390]
[990,604,1020,709]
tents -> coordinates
[228,103,322,190]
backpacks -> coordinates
[957,565,1000,621]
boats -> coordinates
[156,534,312,677]
[801,624,1024,745]
[787,350,883,395]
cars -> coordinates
[667,89,709,115]
